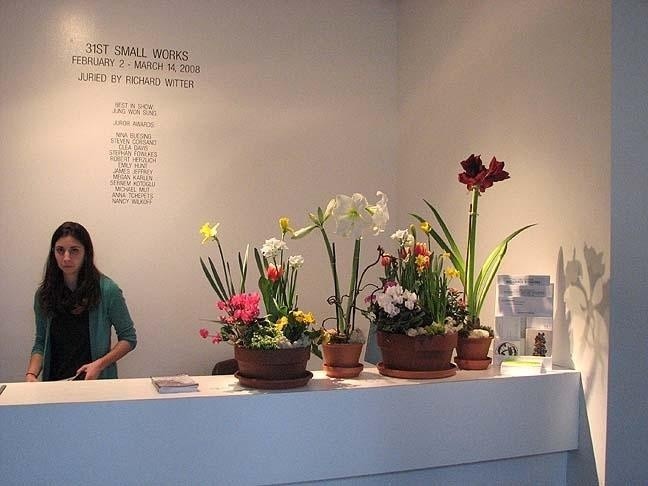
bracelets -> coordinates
[26,372,38,378]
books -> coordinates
[150,373,200,393]
[500,359,543,374]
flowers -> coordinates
[195,217,334,361]
[408,151,540,328]
[291,190,466,346]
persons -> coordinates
[24,220,138,382]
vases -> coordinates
[232,346,313,389]
[374,331,494,380]
[320,344,365,378]
